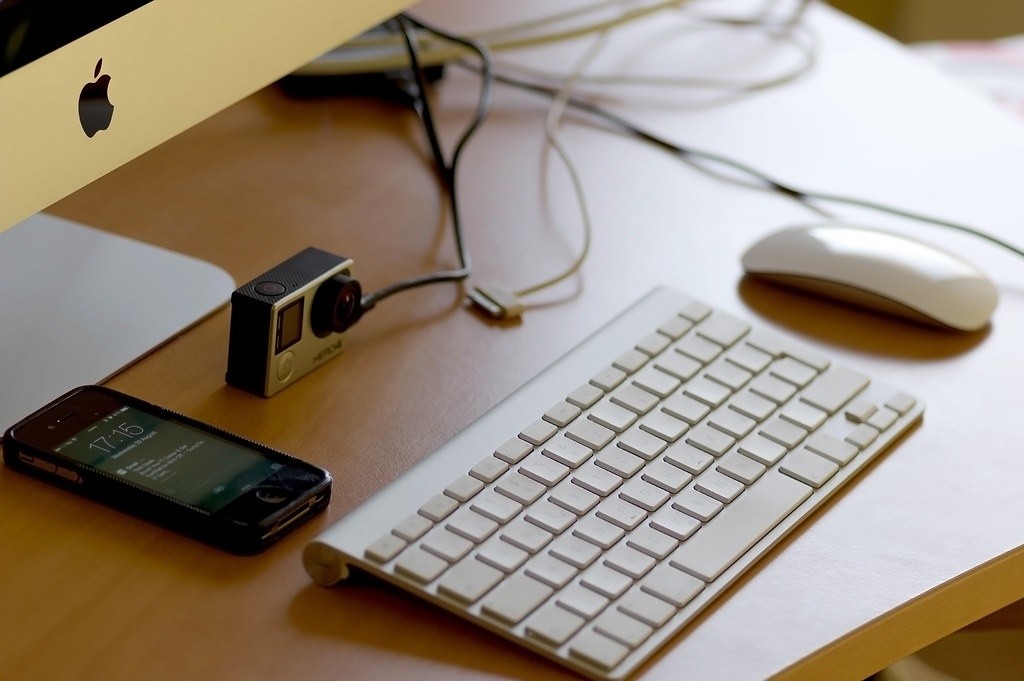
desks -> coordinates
[0,0,1023,681]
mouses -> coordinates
[742,225,999,335]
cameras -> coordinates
[225,246,362,398]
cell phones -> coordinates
[2,385,333,556]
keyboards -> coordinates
[306,287,925,681]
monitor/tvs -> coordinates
[0,0,424,439]
